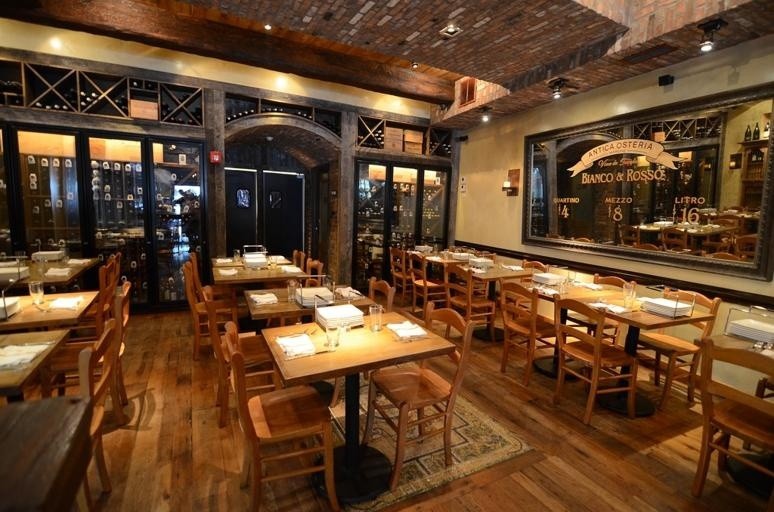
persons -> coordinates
[171,188,201,255]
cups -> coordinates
[287,280,298,304]
[637,206,756,232]
[326,319,341,347]
[432,244,577,297]
[15,247,69,305]
[621,282,636,310]
[368,303,382,333]
[234,248,279,270]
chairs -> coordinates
[533,196,759,262]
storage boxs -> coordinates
[383,124,406,142]
[129,99,161,122]
[652,130,667,143]
[404,130,425,144]
[403,141,423,155]
[384,137,405,153]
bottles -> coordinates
[429,141,453,158]
[359,127,386,148]
[4,68,312,130]
[744,121,770,141]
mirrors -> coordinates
[520,77,773,287]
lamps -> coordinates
[544,74,568,103]
[696,15,725,56]
[479,105,495,123]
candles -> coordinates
[503,179,512,191]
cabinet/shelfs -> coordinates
[0,42,209,144]
[389,179,416,252]
[736,139,772,214]
[632,109,730,155]
[18,149,83,262]
[153,158,213,304]
[419,183,445,247]
[354,107,457,170]
[353,176,385,291]
[85,155,149,304]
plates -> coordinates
[294,285,335,308]
[728,316,774,345]
[644,296,693,317]
[314,303,366,332]
